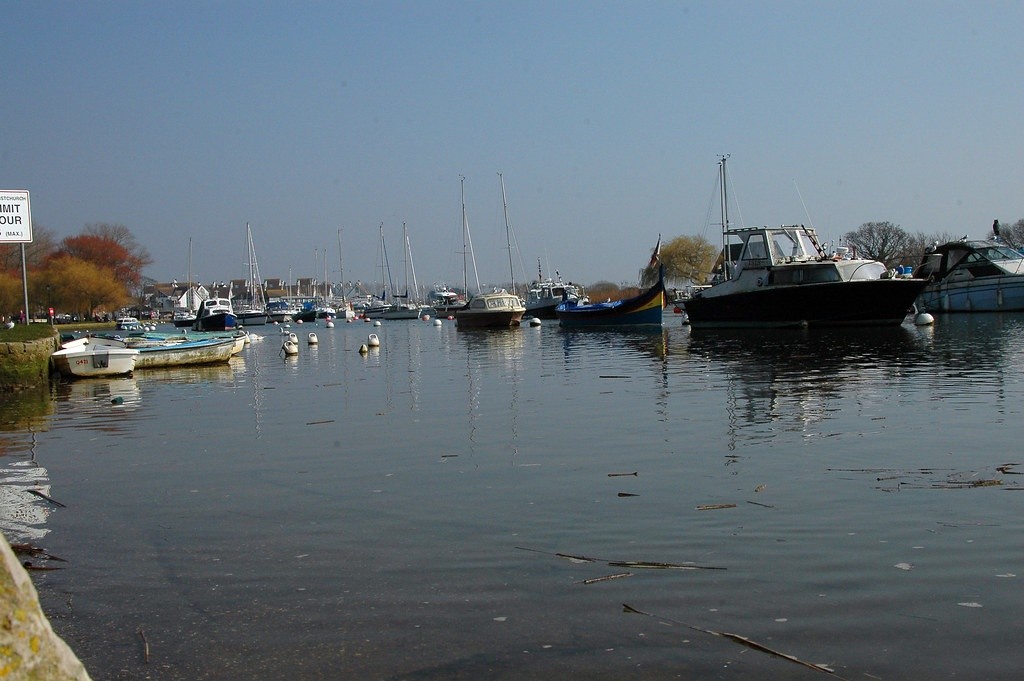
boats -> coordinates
[682,146,929,336]
[195,297,237,332]
[675,295,691,310]
[912,238,1023,312]
[52,331,251,379]
[555,262,668,328]
[520,257,580,317]
[119,318,138,331]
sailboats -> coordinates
[238,169,525,329]
[172,235,198,327]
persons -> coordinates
[18,310,25,324]
[895,264,903,278]
